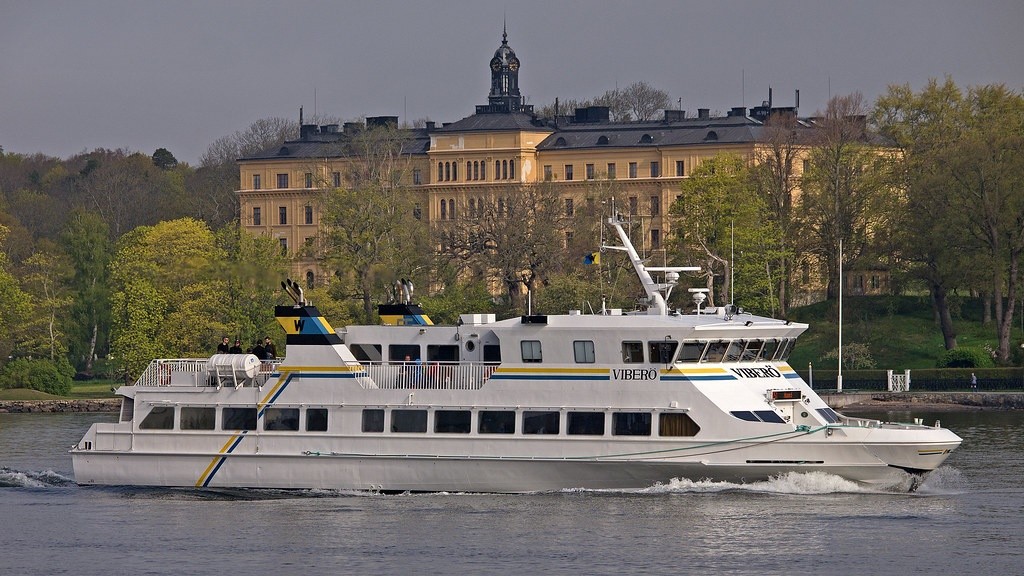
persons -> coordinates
[230,339,242,354]
[217,336,229,354]
[970,373,977,392]
[265,337,276,363]
[396,355,417,388]
[252,340,267,364]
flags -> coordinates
[584,252,600,265]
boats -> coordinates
[67,216,966,495]
